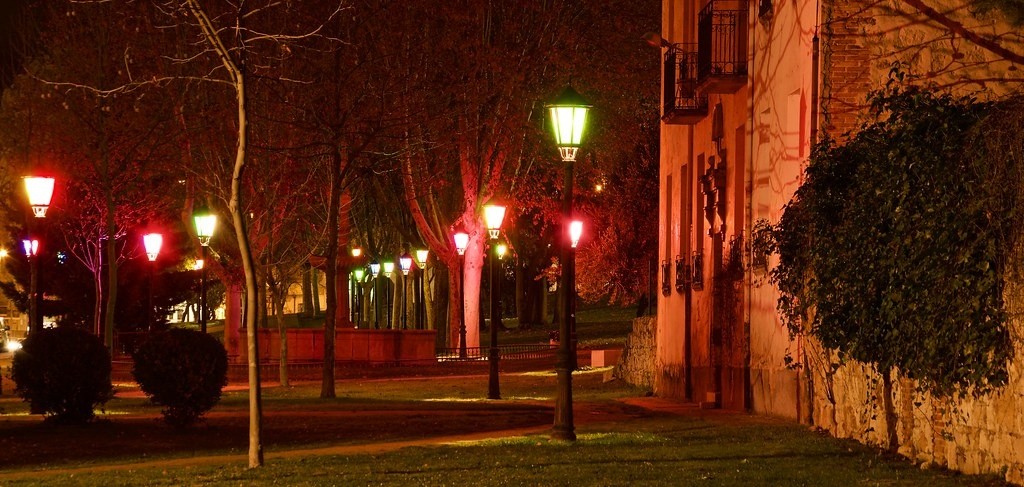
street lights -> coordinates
[399,251,412,329]
[22,173,56,330]
[415,244,430,329]
[481,188,507,400]
[351,245,361,270]
[568,219,584,358]
[495,242,507,320]
[370,259,381,329]
[143,223,163,335]
[192,204,217,332]
[383,258,395,329]
[21,236,39,334]
[452,222,469,359]
[544,75,594,440]
[355,267,364,329]
